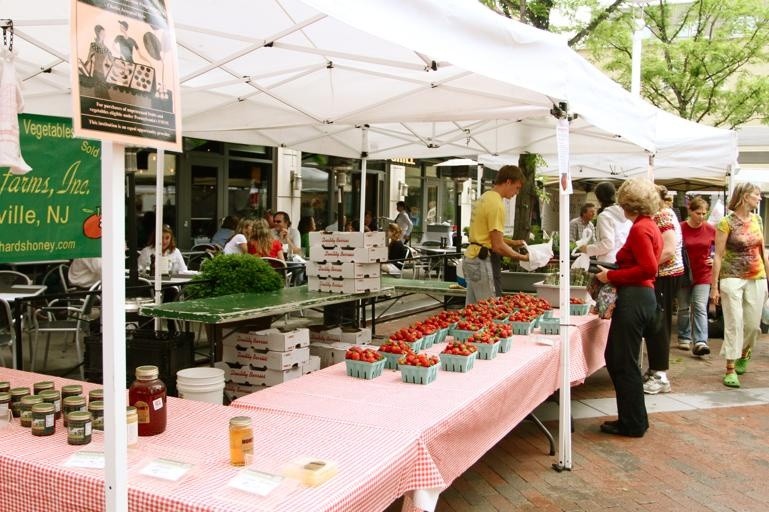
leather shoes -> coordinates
[599,420,645,437]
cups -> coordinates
[287,248,294,261]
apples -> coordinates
[82,206,102,239]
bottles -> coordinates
[440,235,446,249]
[128,364,167,436]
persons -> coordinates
[83,24,114,99]
[462,165,528,307]
[112,20,150,61]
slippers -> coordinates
[722,372,741,387]
[736,347,752,374]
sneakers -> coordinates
[692,340,711,356]
[677,339,691,350]
[642,372,672,394]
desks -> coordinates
[0,369,421,512]
[394,278,612,433]
[228,334,559,457]
[137,276,398,368]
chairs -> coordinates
[387,241,470,281]
[1,242,308,382]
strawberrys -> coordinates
[346,291,586,368]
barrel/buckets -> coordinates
[175,367,226,407]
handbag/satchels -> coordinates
[707,318,724,338]
[587,259,618,275]
[679,244,693,289]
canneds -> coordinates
[61,385,83,417]
[63,395,86,426]
[88,401,104,430]
[66,411,92,445]
[0,381,61,437]
[88,389,104,403]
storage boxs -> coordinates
[306,231,388,279]
